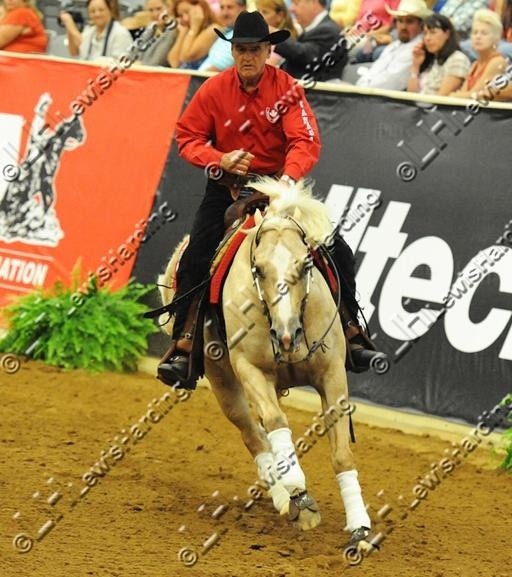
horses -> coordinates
[162,172,379,553]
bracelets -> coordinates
[280,174,296,187]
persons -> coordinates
[156,10,385,391]
[0,0,512,116]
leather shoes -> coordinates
[158,356,196,390]
[347,347,384,370]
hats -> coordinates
[385,0,435,20]
[214,10,291,45]
